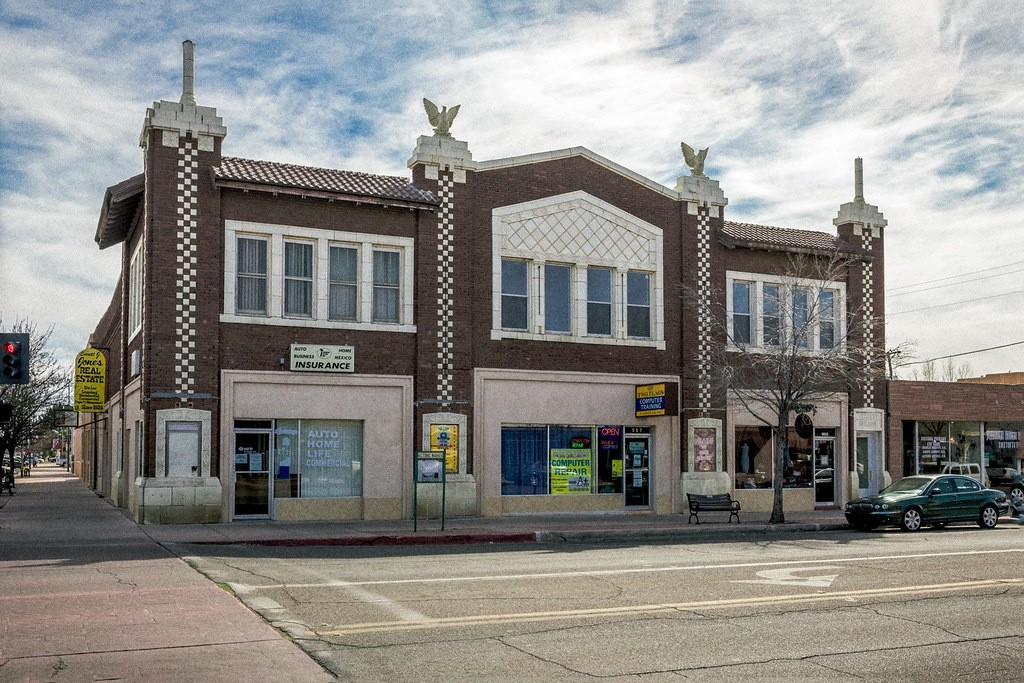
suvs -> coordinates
[921,462,991,488]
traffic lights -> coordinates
[0,333,29,384]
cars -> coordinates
[799,465,834,484]
[1,453,71,475]
[844,474,1011,532]
[1009,475,1024,521]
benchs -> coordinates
[685,493,742,525]
